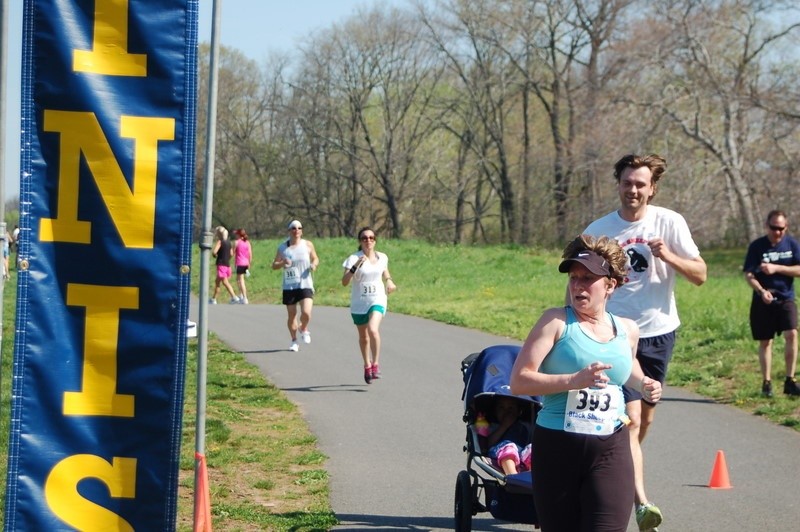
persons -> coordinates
[743,209,800,398]
[561,152,707,532]
[510,235,661,532]
[487,396,533,476]
[342,227,396,384]
[272,219,319,352]
[209,226,251,304]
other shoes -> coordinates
[635,501,662,532]
[298,323,310,344]
[762,380,773,398]
[371,362,381,379]
[783,378,800,396]
[364,366,373,384]
[289,343,299,352]
[230,296,240,304]
[239,295,248,304]
[209,298,217,305]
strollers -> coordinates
[454,345,544,532]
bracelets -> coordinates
[386,278,392,281]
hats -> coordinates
[558,248,613,276]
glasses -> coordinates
[769,225,784,231]
[361,236,375,241]
[291,227,303,229]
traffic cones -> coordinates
[709,450,734,489]
